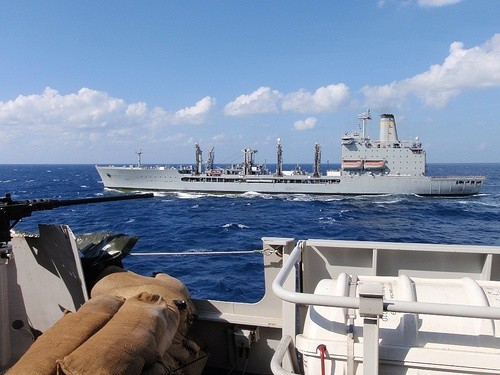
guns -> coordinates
[0,192,154,245]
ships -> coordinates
[93,107,486,197]
